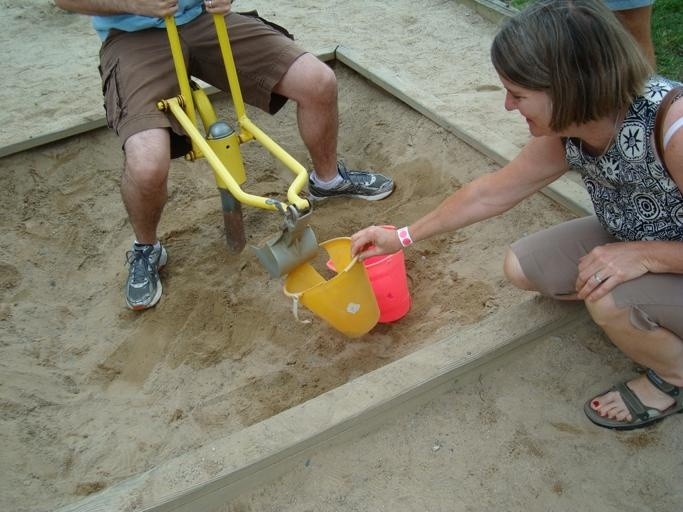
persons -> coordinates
[601,0,656,72]
[53,0,400,315]
[350,0,683,430]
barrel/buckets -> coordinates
[362,226,412,324]
[283,237,380,339]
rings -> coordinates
[594,272,601,283]
[208,1,212,9]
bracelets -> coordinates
[395,224,413,249]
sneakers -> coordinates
[122,240,169,314]
[307,166,397,204]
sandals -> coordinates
[584,369,681,430]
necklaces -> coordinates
[578,109,620,165]
